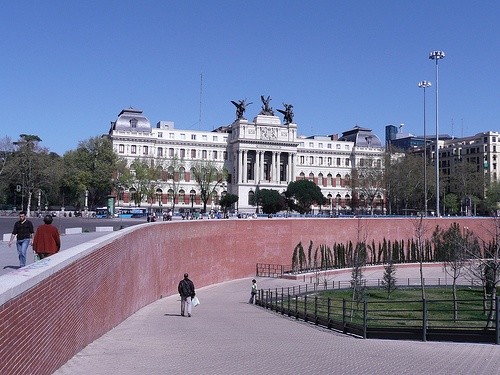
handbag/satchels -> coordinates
[191,296,200,307]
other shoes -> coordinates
[188,312,191,317]
[181,311,184,315]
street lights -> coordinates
[428,51,446,217]
[418,80,432,217]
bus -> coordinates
[95,206,146,219]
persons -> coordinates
[163,209,173,221]
[32,214,61,260]
[98,210,105,215]
[268,213,272,218]
[182,210,229,220]
[35,211,82,217]
[122,210,131,214]
[238,212,257,218]
[248,279,257,304]
[147,213,156,222]
[177,273,196,316]
[9,211,34,268]
